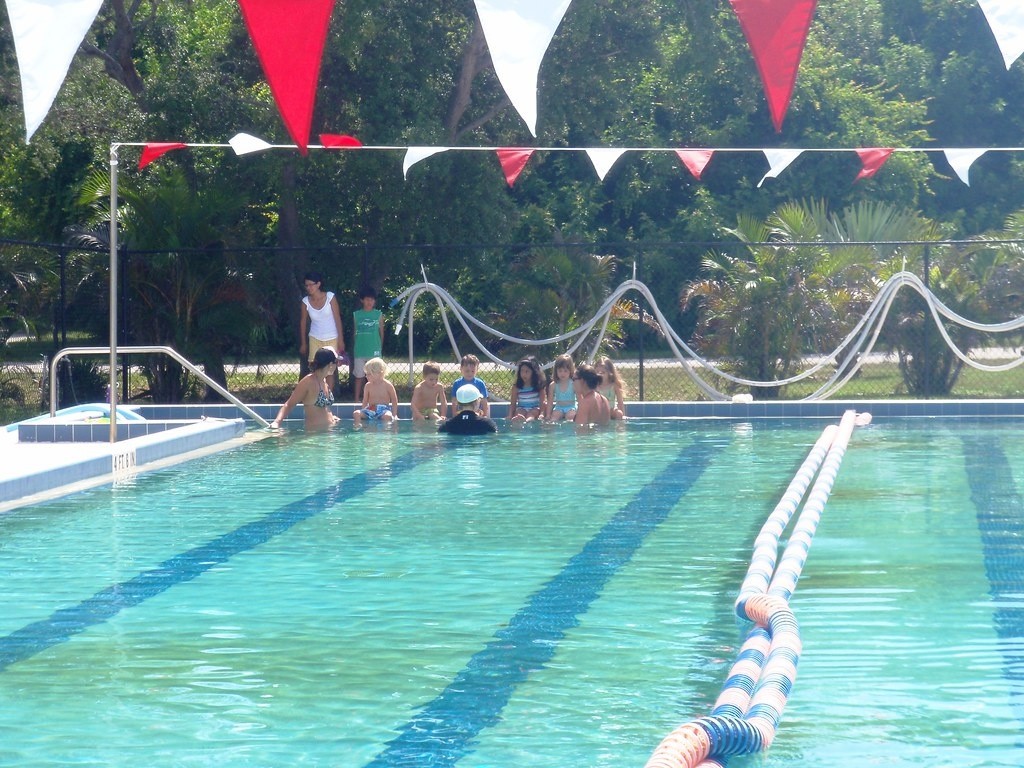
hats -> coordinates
[456,384,483,403]
[315,346,343,362]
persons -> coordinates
[570,364,610,423]
[353,357,399,431]
[436,384,497,435]
[408,360,447,422]
[298,272,345,402]
[450,354,489,418]
[592,356,628,421]
[543,353,583,421]
[263,346,343,430]
[504,354,546,423]
[351,286,385,404]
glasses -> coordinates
[572,377,581,382]
